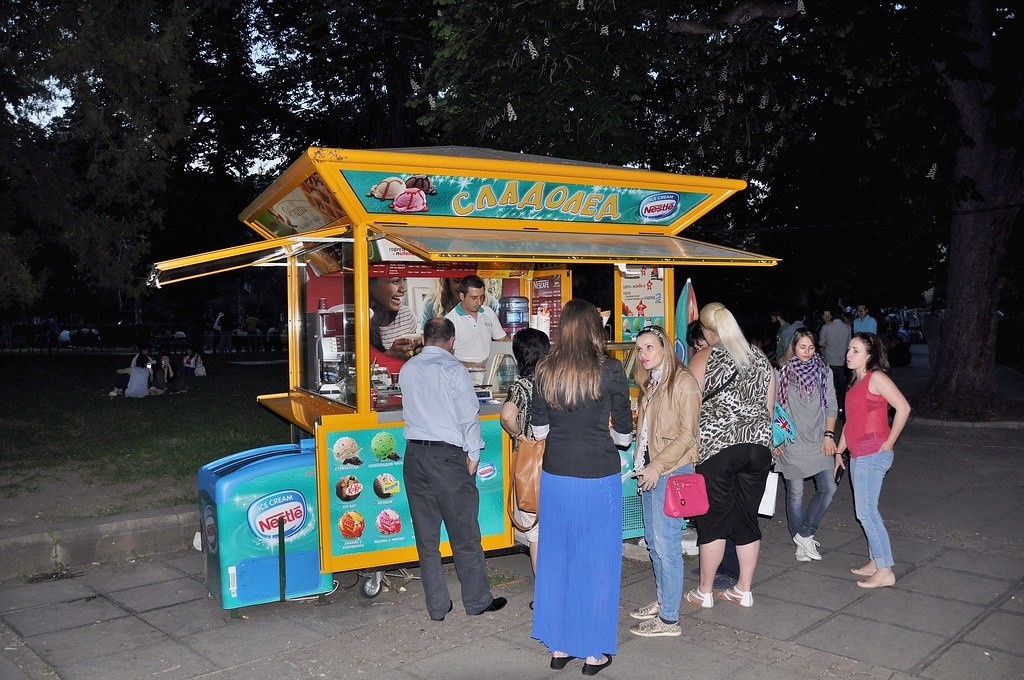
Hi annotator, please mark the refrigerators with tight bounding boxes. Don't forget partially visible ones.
[198,443,333,618]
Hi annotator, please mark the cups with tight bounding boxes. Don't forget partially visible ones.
[391,373,399,389]
[371,393,378,410]
[531,304,551,344]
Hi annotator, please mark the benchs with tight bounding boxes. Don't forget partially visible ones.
[154,333,275,356]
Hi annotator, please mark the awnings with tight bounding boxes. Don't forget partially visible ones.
[146,223,353,288]
[367,222,782,267]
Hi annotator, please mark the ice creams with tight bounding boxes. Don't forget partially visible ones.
[332,432,401,538]
[622,317,661,332]
[366,172,437,212]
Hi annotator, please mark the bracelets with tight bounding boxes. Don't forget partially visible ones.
[834,452,842,455]
[824,430,835,438]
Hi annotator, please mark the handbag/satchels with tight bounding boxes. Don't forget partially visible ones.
[505,437,548,530]
[757,470,779,517]
[663,474,715,517]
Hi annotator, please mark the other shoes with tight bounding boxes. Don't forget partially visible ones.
[474,596,510,616]
[851,567,881,577]
[549,654,579,669]
[529,601,536,612]
[581,652,613,676]
[430,599,453,621]
[857,572,896,590]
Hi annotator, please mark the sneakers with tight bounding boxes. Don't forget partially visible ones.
[629,616,681,637]
[795,545,815,562]
[628,601,663,619]
[792,533,823,561]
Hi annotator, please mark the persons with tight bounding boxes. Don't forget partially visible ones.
[529,298,633,676]
[115,343,207,398]
[499,327,554,611]
[421,278,465,346]
[819,305,852,423]
[767,305,877,371]
[173,328,185,339]
[58,326,100,351]
[211,310,261,354]
[881,305,911,369]
[398,317,508,621]
[442,274,513,386]
[368,277,425,357]
[683,302,776,610]
[773,326,839,561]
[833,331,911,589]
[629,324,704,637]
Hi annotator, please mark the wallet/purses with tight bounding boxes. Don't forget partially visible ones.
[834,458,849,485]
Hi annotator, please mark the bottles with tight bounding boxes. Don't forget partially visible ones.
[370,364,388,384]
[498,296,529,342]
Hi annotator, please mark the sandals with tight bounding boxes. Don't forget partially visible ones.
[717,586,755,607]
[684,586,716,608]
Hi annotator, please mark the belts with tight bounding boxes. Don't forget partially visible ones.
[407,439,456,449]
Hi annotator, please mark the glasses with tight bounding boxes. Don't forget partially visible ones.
[857,308,866,312]
